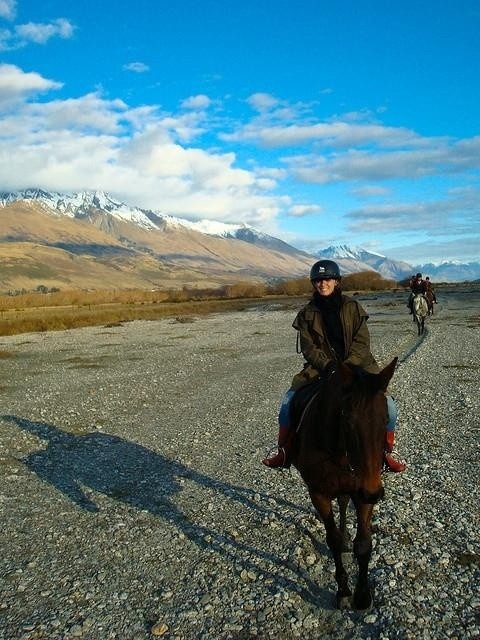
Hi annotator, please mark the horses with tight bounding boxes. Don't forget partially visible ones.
[425,286,435,316]
[289,356,398,615]
[412,295,428,337]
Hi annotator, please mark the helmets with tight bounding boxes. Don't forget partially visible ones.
[308,259,341,279]
[416,272,422,278]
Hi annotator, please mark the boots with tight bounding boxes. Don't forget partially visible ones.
[385,431,408,474]
[262,427,292,469]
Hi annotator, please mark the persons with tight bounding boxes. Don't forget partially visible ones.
[408,273,439,316]
[263,260,406,473]
